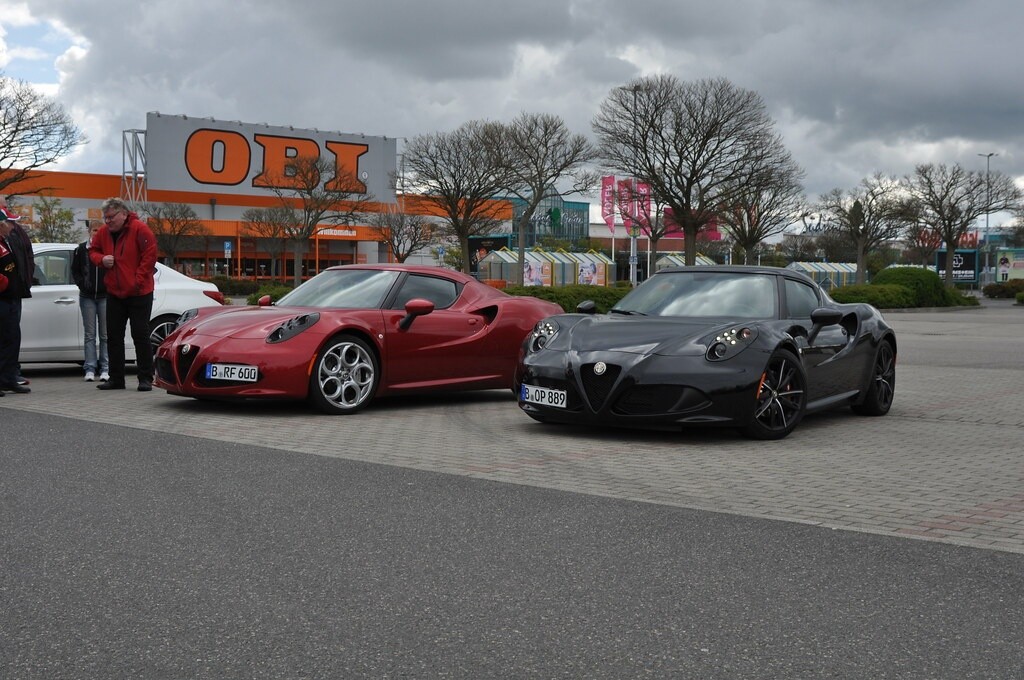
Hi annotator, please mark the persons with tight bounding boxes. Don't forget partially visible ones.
[72,220,111,382]
[88,198,156,391]
[998,253,1010,282]
[0,196,34,395]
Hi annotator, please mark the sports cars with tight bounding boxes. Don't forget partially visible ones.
[152,264,566,414]
[516,265,897,441]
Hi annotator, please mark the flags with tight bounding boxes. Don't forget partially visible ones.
[601,175,651,236]
[718,202,758,228]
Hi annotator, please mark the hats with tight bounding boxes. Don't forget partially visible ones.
[0,207,21,221]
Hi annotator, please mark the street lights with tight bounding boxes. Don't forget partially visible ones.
[619,84,650,286]
[978,152,994,287]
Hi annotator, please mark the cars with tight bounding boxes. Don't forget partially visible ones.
[20,241,225,371]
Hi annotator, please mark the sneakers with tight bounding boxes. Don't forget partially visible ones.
[16,376,29,385]
[85,372,94,381]
[99,372,110,381]
[0,382,30,394]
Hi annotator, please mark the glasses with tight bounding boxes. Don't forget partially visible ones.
[103,212,119,220]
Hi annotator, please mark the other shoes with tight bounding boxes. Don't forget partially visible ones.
[137,380,152,391]
[96,377,126,389]
